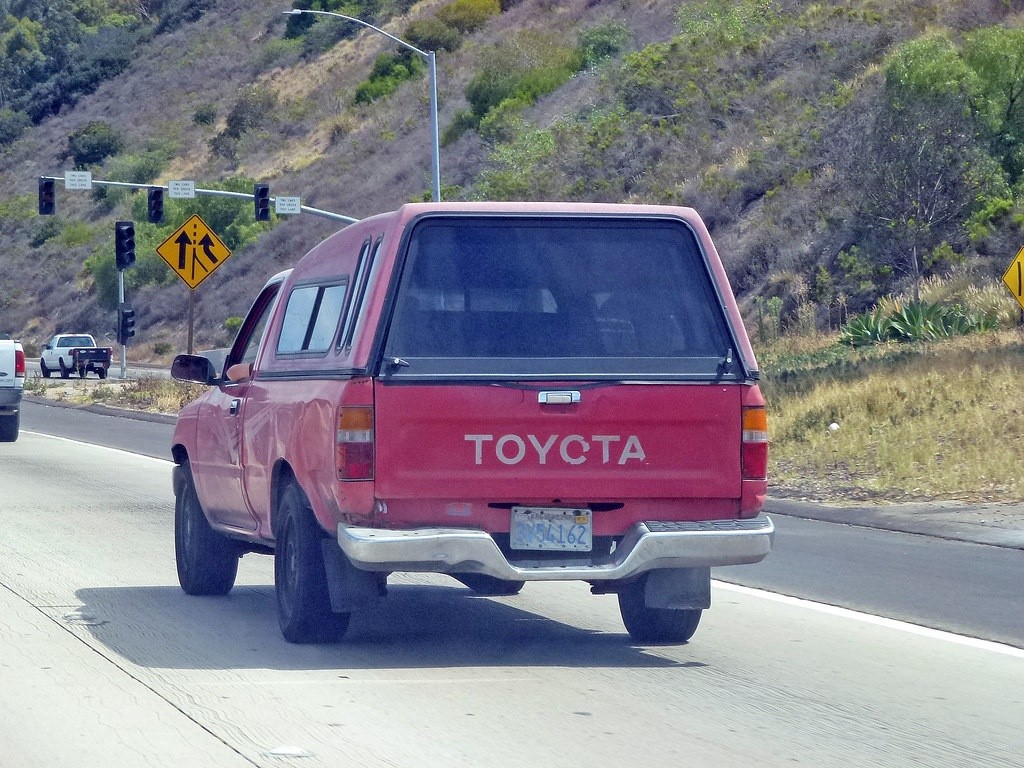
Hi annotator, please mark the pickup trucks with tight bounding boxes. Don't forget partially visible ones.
[40,333,114,379]
[0,339,27,442]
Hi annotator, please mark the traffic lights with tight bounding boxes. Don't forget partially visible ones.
[253,184,270,221]
[115,220,136,272]
[148,187,163,224]
[38,177,56,216]
[117,307,135,346]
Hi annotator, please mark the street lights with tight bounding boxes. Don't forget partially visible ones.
[280,7,441,203]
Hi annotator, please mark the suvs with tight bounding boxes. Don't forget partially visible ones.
[172,198,772,646]
